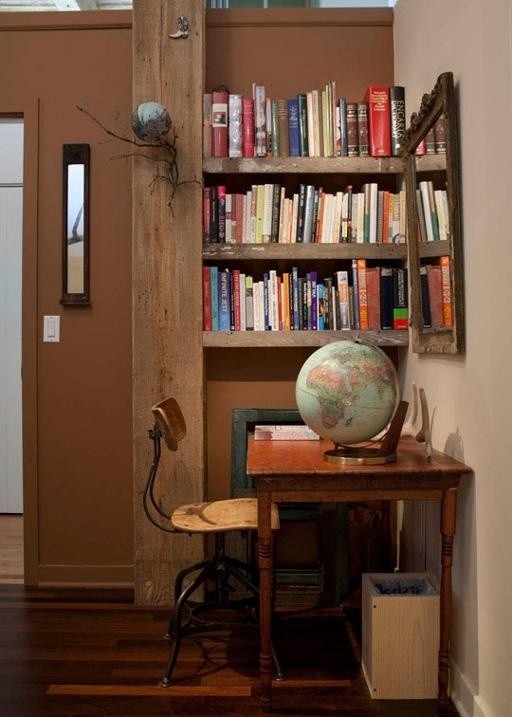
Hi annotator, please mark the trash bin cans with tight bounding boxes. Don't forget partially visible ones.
[359,571,440,700]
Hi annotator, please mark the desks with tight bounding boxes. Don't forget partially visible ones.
[243,435,474,712]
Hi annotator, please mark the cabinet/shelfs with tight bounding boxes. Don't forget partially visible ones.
[413,147,452,340]
[198,150,411,352]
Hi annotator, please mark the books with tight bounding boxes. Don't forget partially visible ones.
[203,258,408,333]
[434,113,446,153]
[204,184,407,244]
[254,424,320,441]
[415,180,452,329]
[204,82,406,158]
[424,126,436,154]
[415,138,425,155]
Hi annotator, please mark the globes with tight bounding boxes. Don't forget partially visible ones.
[295,333,409,465]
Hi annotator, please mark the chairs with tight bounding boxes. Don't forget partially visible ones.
[138,392,293,690]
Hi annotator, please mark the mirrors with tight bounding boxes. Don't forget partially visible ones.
[400,69,467,353]
